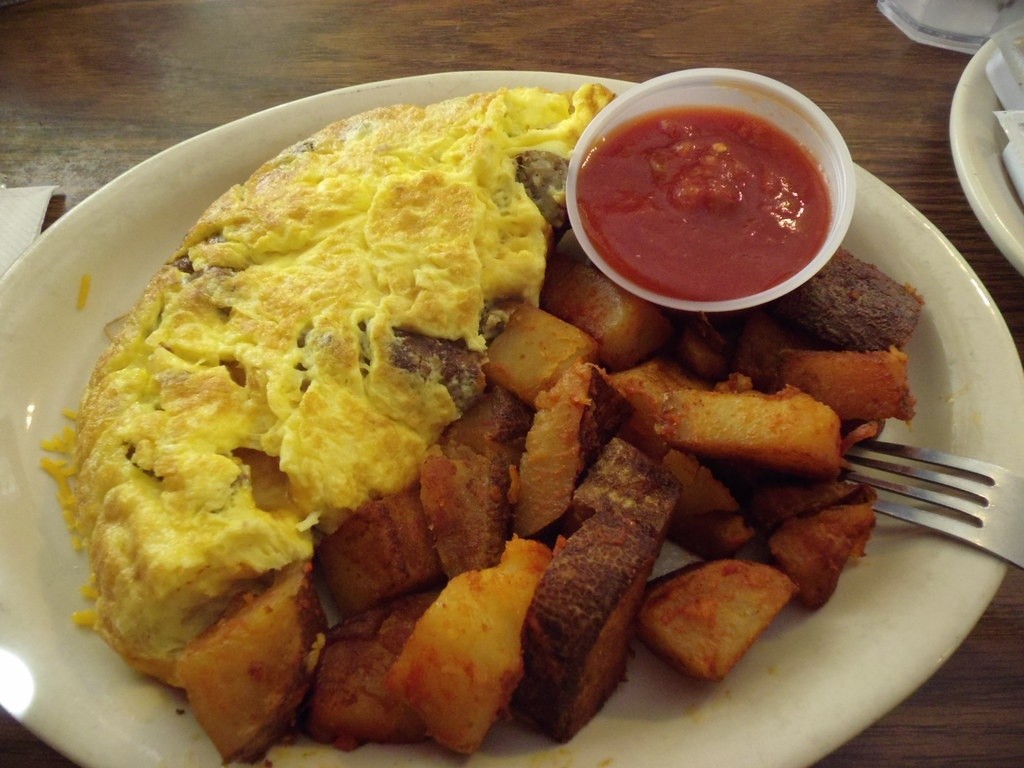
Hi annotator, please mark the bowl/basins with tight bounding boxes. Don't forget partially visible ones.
[564,67,861,314]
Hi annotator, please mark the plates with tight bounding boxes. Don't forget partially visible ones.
[0,68,1023,768]
[947,17,1023,280]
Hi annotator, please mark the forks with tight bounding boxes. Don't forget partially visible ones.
[836,438,1024,572]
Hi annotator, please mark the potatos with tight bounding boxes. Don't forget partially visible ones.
[140,246,926,768]
